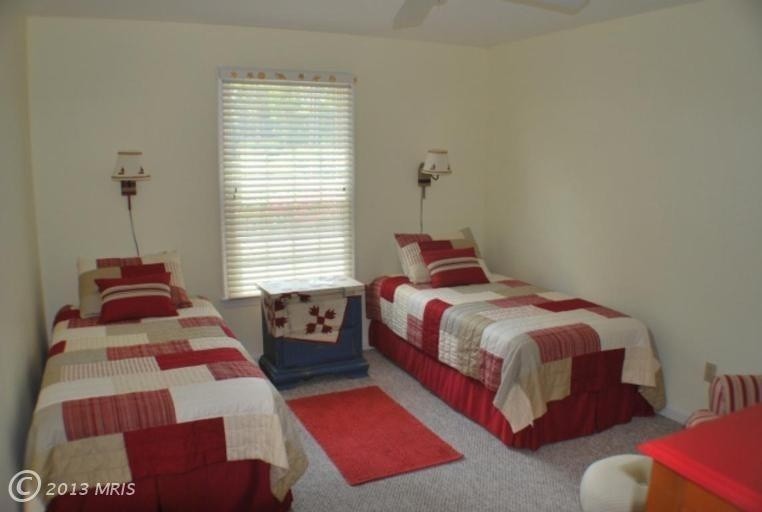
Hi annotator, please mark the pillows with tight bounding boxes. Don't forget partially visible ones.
[394,228,493,288]
[76,248,192,323]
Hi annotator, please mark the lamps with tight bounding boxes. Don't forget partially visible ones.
[416,149,454,235]
[109,151,150,257]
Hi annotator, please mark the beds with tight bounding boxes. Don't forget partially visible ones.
[363,271,668,452]
[21,296,309,512]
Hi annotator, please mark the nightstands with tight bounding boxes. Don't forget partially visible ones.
[253,273,368,392]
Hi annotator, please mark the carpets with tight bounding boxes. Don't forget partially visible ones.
[285,384,465,488]
